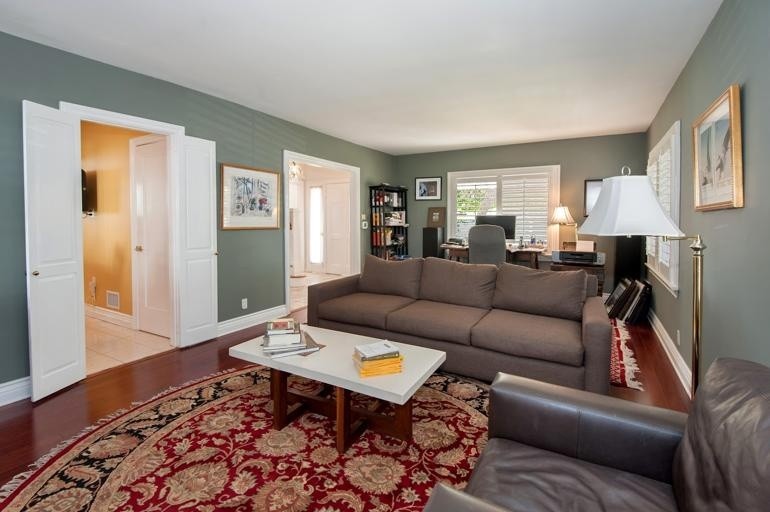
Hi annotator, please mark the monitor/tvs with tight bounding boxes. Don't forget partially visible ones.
[475,215,516,240]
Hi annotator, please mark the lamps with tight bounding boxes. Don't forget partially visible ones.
[575,165,706,399]
[549,204,575,227]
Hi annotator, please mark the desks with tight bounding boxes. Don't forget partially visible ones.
[439,241,545,269]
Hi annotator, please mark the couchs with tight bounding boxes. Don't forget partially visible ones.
[421,356,770,512]
[307,253,612,396]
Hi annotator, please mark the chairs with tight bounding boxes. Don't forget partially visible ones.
[467,224,506,264]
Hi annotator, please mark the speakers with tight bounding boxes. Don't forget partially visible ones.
[422,227,444,258]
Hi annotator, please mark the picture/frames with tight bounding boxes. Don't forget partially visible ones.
[219,162,281,230]
[690,82,745,211]
[414,176,442,201]
[582,178,604,217]
[606,277,653,324]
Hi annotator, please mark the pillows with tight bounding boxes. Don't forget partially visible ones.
[418,257,498,310]
[492,261,587,319]
[358,253,424,300]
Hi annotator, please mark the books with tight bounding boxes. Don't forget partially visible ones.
[352,340,404,378]
[259,318,328,359]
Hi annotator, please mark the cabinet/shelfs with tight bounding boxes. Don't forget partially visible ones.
[549,263,608,297]
[368,185,408,260]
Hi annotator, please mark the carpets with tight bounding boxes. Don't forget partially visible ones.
[0,359,489,512]
[609,317,644,392]
